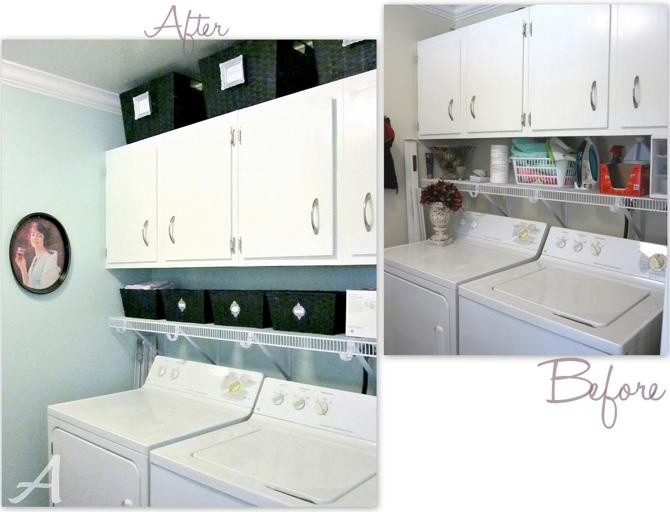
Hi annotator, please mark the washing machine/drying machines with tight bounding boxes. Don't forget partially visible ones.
[384,210,547,356]
[147,375,377,509]
[45,355,264,508]
[457,224,669,357]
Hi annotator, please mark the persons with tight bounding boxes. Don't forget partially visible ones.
[14,222,60,289]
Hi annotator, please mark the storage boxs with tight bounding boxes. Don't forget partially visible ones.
[120,288,164,319]
[160,289,213,324]
[312,39,376,84]
[207,291,271,328]
[266,291,346,335]
[198,40,316,119]
[118,72,206,144]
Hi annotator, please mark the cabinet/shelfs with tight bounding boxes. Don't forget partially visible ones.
[105,71,378,271]
[419,3,669,141]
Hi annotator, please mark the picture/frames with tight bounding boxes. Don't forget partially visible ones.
[8,212,71,295]
